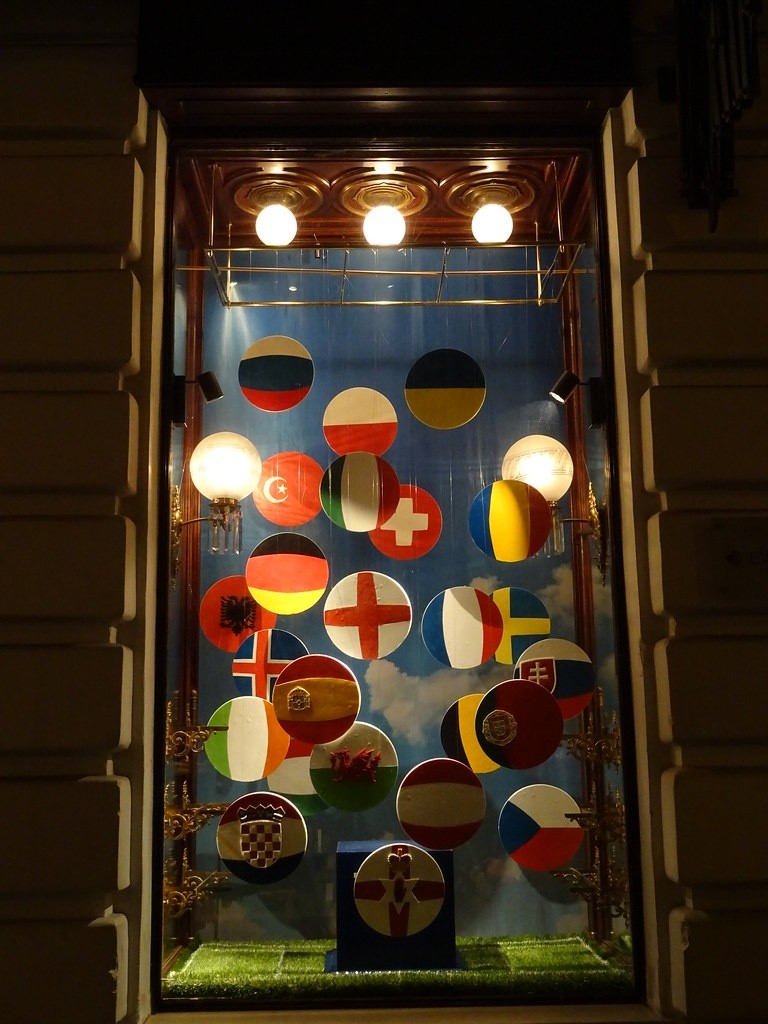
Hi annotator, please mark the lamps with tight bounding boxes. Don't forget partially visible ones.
[549,371,604,430]
[172,371,223,427]
[501,434,607,585]
[169,431,263,594]
